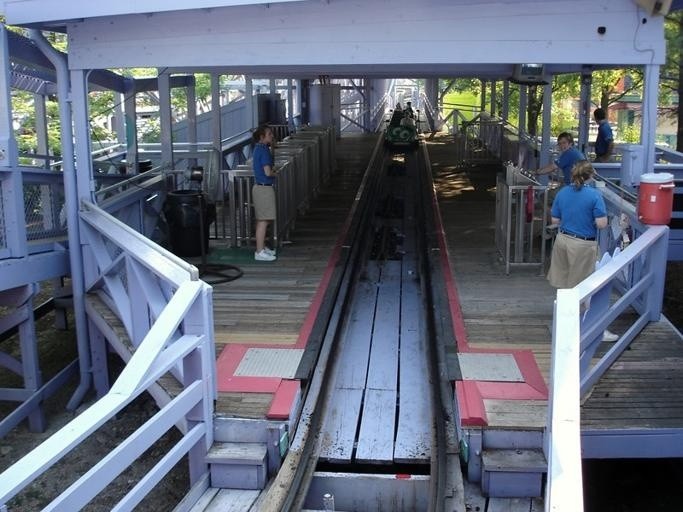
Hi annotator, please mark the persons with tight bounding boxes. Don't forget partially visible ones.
[394,102,416,127]
[546,161,620,343]
[590,108,616,162]
[529,133,589,186]
[251,126,277,263]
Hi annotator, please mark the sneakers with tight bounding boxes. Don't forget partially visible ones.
[264,246,275,255]
[601,330,618,342]
[255,249,276,261]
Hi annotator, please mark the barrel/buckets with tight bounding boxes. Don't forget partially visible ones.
[162,189,216,258]
[638,172,676,226]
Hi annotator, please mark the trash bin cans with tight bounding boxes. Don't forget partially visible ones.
[166,189,209,257]
[119,158,153,199]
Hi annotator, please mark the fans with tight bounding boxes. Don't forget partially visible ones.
[184,148,243,285]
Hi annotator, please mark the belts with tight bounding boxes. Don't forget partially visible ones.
[258,184,272,186]
[561,230,595,240]
